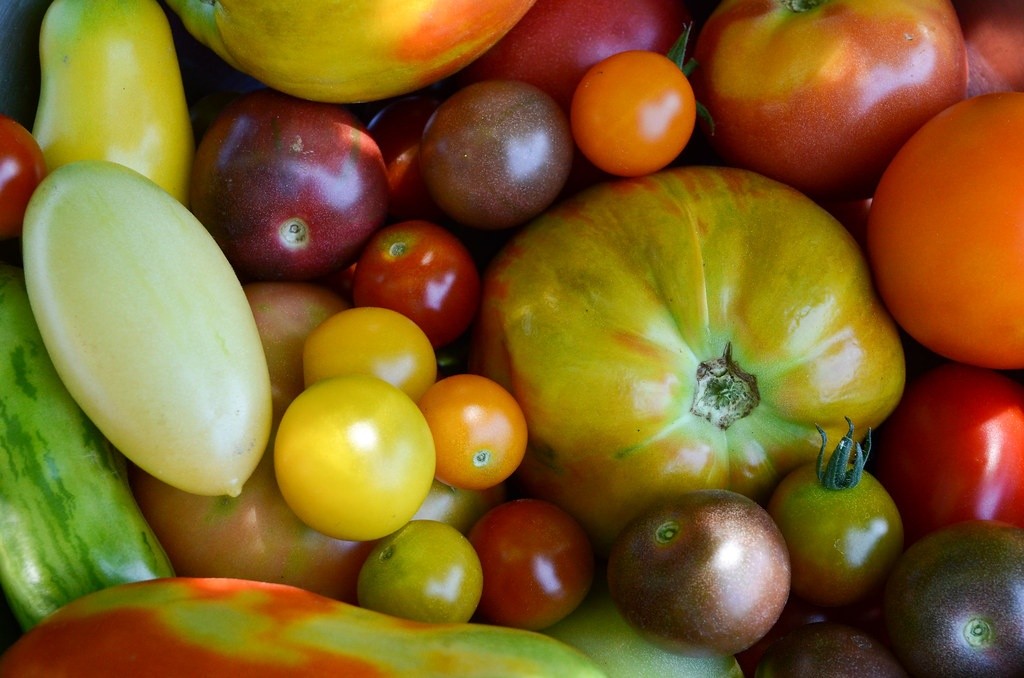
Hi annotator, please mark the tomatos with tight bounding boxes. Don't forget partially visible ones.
[0,0,1024,678]
